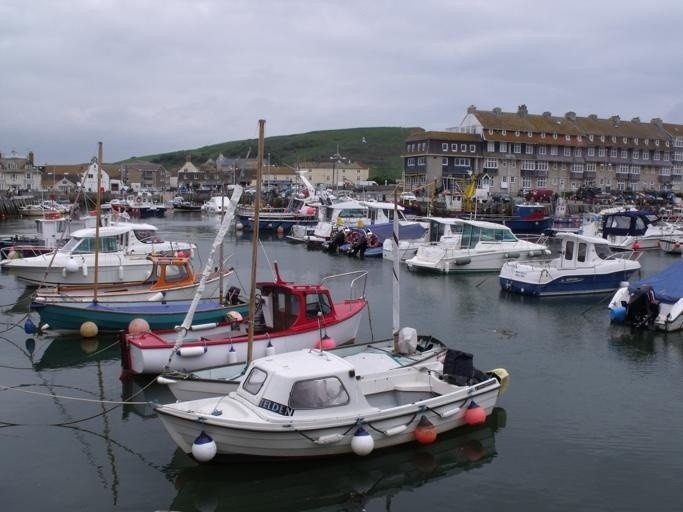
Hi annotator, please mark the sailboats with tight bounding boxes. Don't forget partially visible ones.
[153,117,512,463]
[24,141,256,338]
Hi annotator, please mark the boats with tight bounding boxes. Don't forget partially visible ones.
[0,167,200,282]
[498,202,560,238]
[116,181,372,378]
[30,240,235,305]
[497,231,641,300]
[604,251,683,335]
[581,206,682,257]
[403,216,552,275]
[199,196,232,215]
[232,178,407,260]
